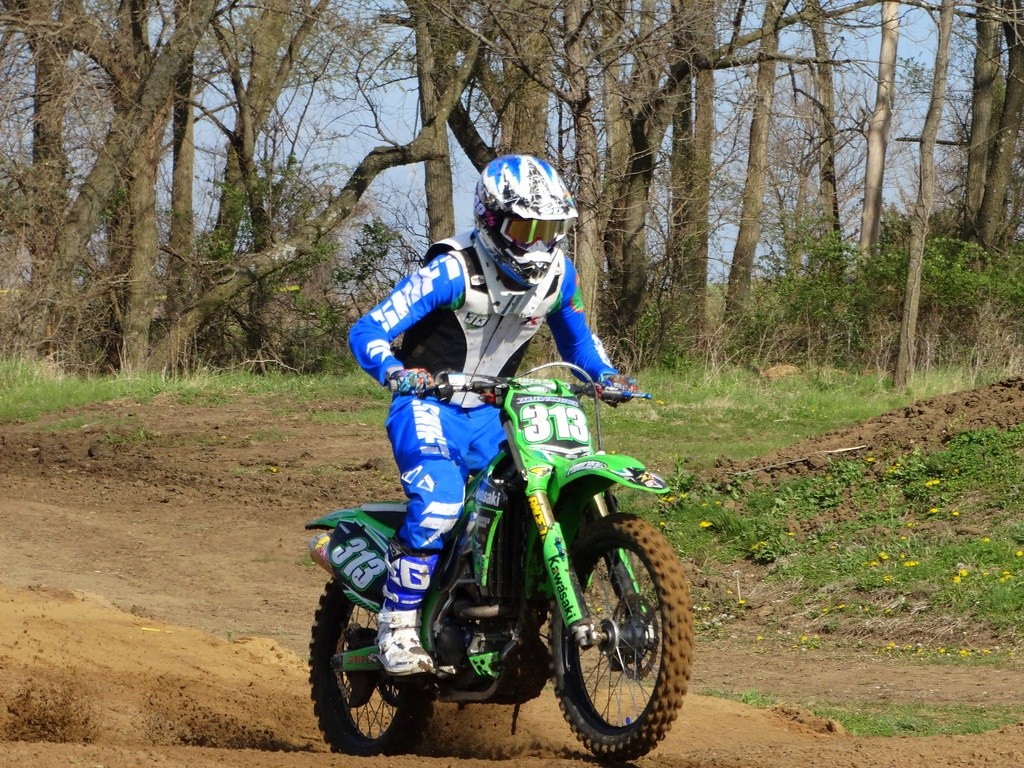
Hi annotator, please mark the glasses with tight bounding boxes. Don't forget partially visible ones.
[484,213,574,253]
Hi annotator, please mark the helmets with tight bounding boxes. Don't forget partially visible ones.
[474,154,579,288]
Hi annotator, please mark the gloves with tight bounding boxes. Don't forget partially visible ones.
[388,368,435,400]
[597,374,638,409]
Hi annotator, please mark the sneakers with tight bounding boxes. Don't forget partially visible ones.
[378,598,433,676]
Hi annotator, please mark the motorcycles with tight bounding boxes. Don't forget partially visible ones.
[307,371,696,766]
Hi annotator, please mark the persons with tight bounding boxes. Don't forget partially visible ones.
[349,154,637,677]
